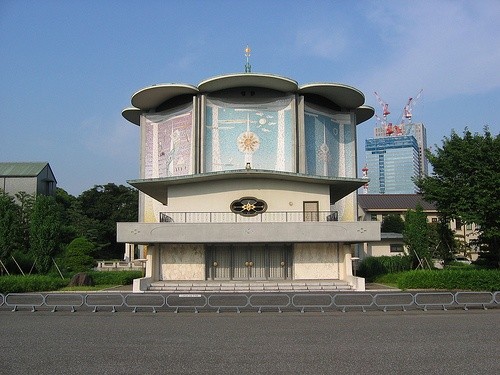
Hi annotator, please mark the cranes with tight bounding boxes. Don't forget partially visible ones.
[373,90,424,137]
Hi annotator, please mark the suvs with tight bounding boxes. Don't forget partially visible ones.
[454,254,471,264]
